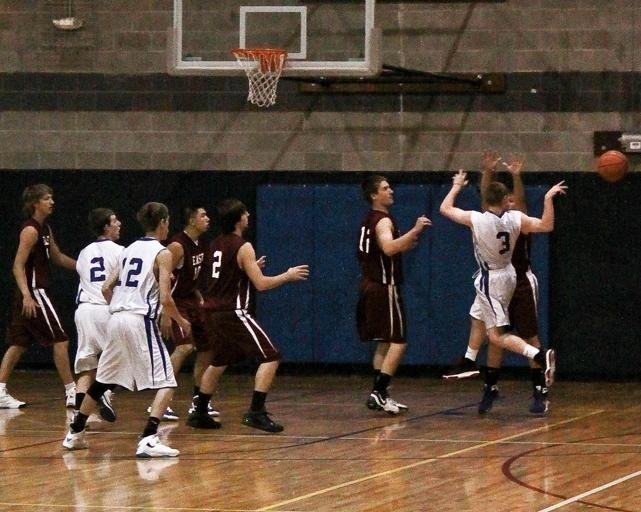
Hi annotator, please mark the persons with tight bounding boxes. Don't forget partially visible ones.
[478,148,551,417]
[185,196,309,432]
[62,201,191,457]
[356,175,432,415]
[0,184,78,410]
[439,168,569,388]
[69,208,126,426]
[146,205,220,420]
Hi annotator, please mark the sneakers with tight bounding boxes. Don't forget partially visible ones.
[368,390,408,416]
[186,397,221,416]
[0,383,27,408]
[530,393,553,414]
[99,389,117,421]
[445,363,480,381]
[239,412,285,432]
[538,349,556,385]
[134,438,182,459]
[477,385,499,415]
[64,387,79,409]
[146,401,178,420]
[63,427,91,449]
[189,412,222,429]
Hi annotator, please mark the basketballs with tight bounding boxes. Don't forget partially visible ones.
[597,150,629,183]
